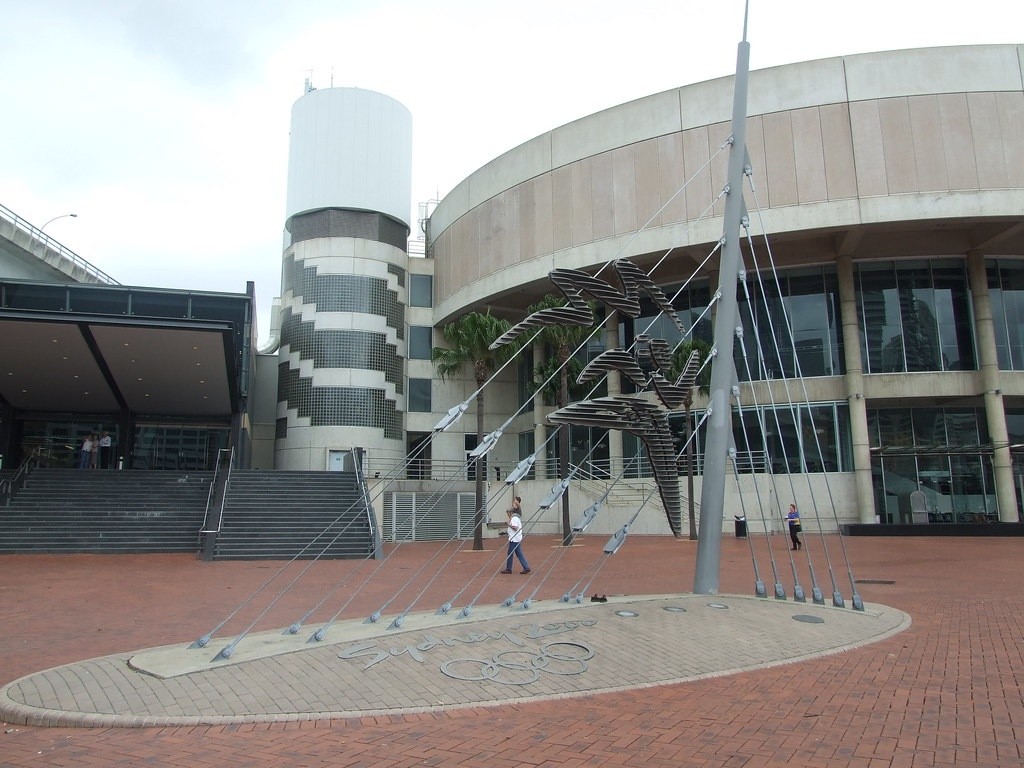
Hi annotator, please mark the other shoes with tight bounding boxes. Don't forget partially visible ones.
[790,547,797,550]
[501,569,511,574]
[520,569,530,574]
[798,543,801,550]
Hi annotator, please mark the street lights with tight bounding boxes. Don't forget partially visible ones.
[36,214,78,245]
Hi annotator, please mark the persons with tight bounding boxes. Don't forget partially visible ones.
[500,497,530,574]
[80,432,112,469]
[785,504,801,550]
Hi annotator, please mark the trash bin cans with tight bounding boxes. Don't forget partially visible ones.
[735,515,747,539]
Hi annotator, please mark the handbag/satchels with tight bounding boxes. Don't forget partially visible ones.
[794,524,802,532]
[79,440,86,450]
